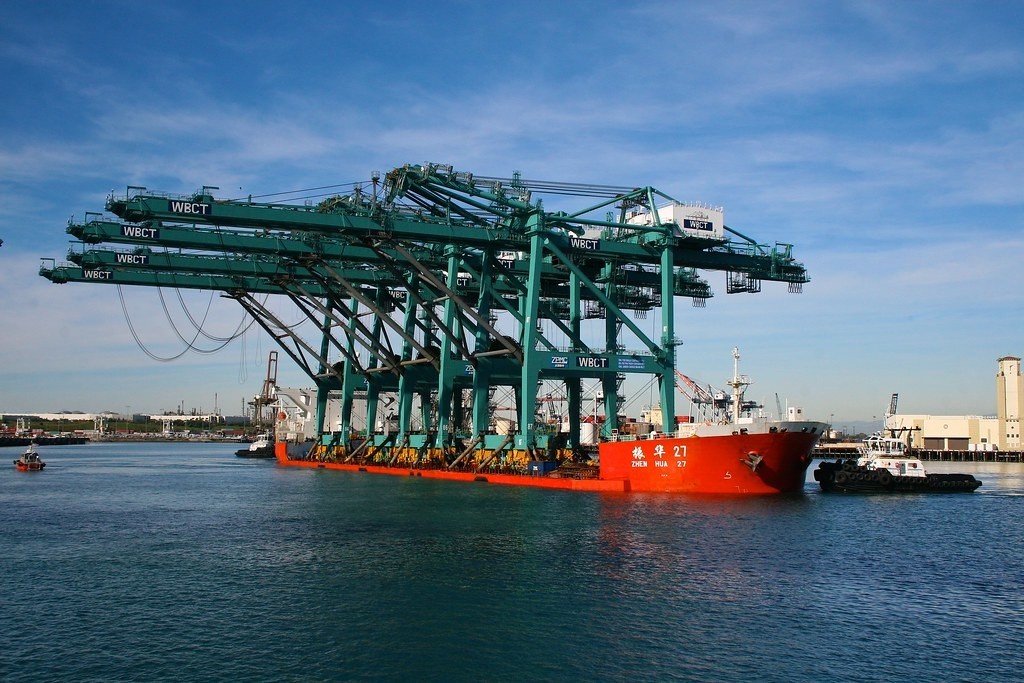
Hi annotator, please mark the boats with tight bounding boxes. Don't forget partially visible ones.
[236,433,276,457]
[14,442,45,472]
[814,427,982,494]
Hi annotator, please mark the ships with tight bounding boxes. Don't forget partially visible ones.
[38,163,830,498]
